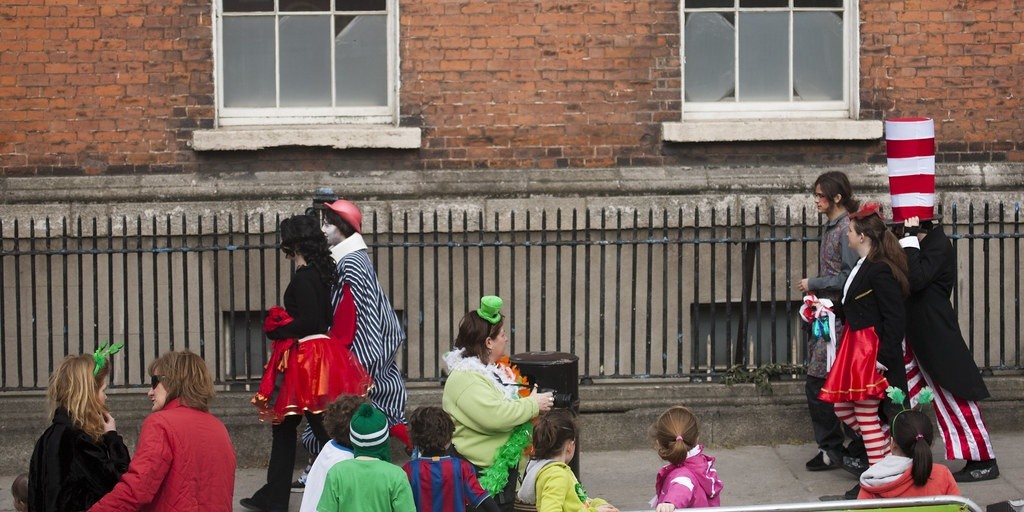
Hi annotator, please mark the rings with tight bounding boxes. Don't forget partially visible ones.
[549,398,553,403]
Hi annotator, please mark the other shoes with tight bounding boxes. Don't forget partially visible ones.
[951,462,1000,482]
[240,498,260,510]
[846,483,861,500]
[806,451,839,470]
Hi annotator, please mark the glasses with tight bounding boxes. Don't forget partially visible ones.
[152,375,166,389]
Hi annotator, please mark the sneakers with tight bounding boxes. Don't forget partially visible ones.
[292,473,307,492]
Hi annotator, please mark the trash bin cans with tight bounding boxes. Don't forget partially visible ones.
[510,352,580,483]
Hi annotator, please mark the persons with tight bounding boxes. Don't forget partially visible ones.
[798,170,861,471]
[402,406,499,512]
[441,295,554,512]
[299,394,369,512]
[517,411,621,512]
[817,202,912,500]
[316,402,416,512]
[238,207,376,512]
[26,340,131,512]
[855,410,962,499]
[885,198,1000,483]
[12,473,30,512]
[86,350,236,511]
[647,406,724,512]
[290,199,412,493]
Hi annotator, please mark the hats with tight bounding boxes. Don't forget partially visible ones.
[350,403,391,461]
[324,200,361,233]
[885,117,943,225]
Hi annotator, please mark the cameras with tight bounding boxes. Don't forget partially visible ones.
[530,385,573,409]
